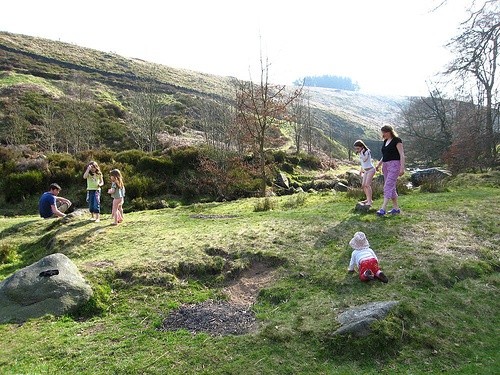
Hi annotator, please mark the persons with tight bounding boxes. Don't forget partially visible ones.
[353,140,376,206]
[83,161,104,223]
[375,125,405,216]
[107,169,125,225]
[348,232,388,283]
[38,183,72,219]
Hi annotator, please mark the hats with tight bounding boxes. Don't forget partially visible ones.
[349,232,369,249]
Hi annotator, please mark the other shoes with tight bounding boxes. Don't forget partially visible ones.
[364,269,374,281]
[90,218,96,220]
[95,219,100,222]
[377,270,388,283]
[361,200,368,203]
[111,222,117,224]
[118,219,123,223]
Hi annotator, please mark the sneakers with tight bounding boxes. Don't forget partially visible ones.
[376,209,386,215]
[387,208,400,214]
[366,200,373,205]
